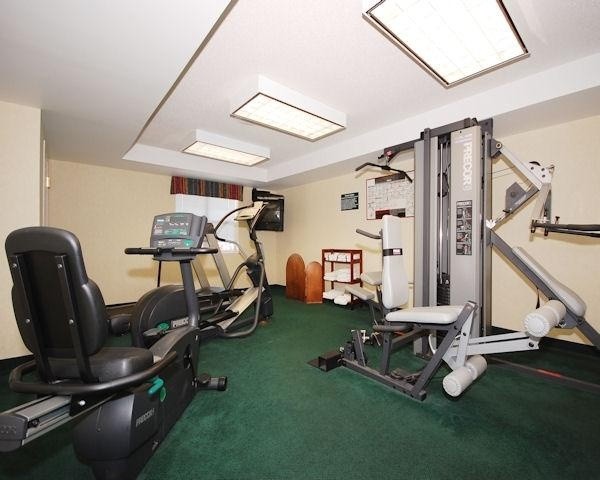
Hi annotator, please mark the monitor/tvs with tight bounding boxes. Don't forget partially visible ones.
[254,198,284,232]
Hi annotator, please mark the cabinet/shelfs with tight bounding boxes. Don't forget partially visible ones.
[322,248,363,309]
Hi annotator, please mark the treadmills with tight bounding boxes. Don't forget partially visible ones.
[105,224,237,339]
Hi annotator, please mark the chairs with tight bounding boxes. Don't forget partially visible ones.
[4,227,152,382]
[382,213,466,325]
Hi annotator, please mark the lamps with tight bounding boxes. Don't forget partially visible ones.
[361,0,530,89]
[228,74,347,144]
[181,130,272,168]
